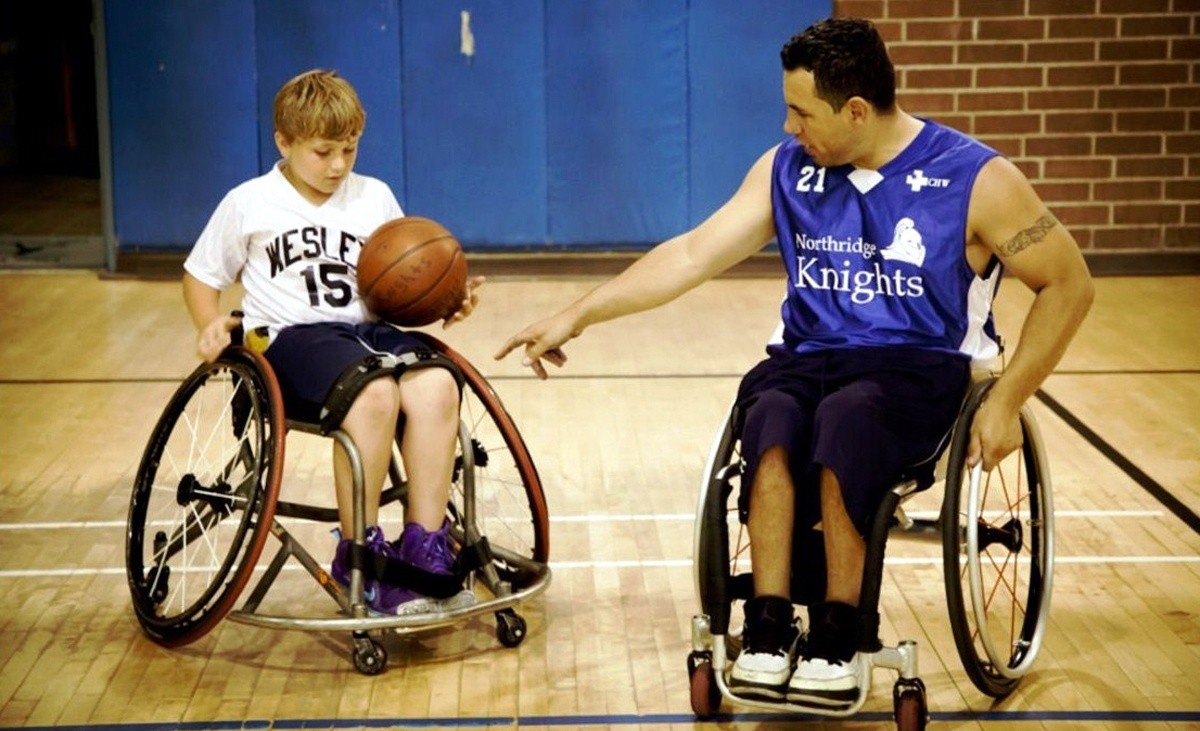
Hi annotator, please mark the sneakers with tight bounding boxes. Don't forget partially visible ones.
[332,525,426,615]
[787,602,863,704]
[732,595,801,686]
[388,518,473,610]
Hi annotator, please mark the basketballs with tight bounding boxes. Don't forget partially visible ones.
[357,216,466,330]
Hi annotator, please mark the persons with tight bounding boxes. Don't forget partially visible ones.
[183,68,488,617]
[493,15,1098,718]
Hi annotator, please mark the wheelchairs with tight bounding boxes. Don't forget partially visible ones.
[686,332,1056,731]
[124,310,552,675]
